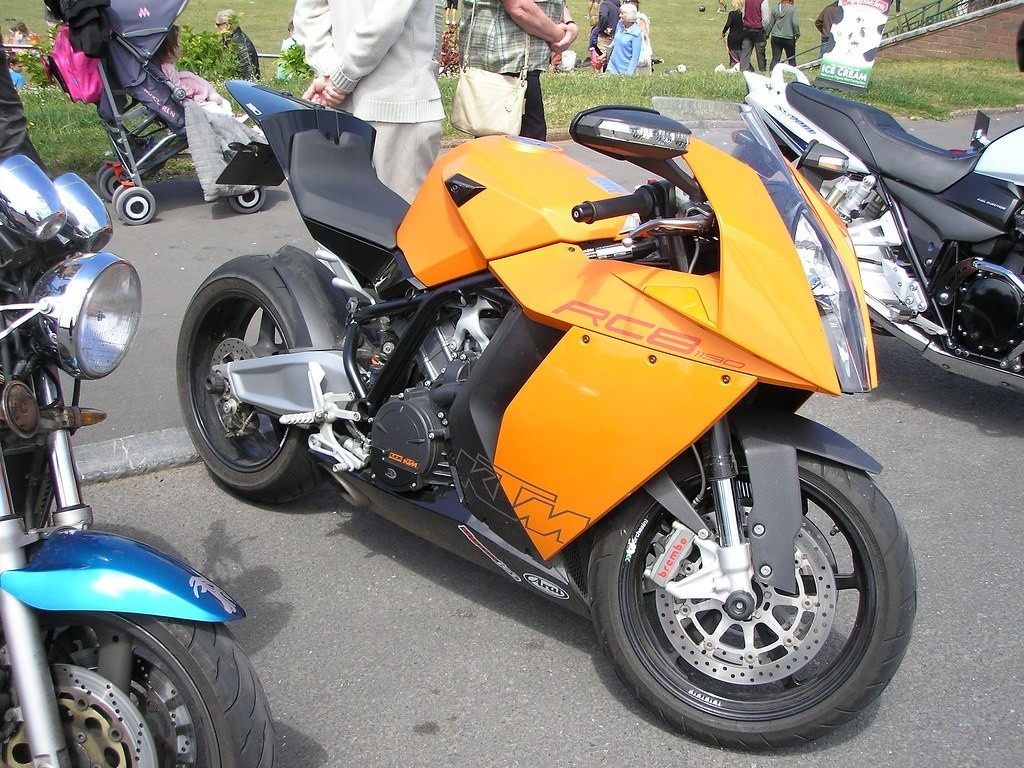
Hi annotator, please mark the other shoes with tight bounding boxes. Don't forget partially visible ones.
[446,20,450,24]
[452,21,456,25]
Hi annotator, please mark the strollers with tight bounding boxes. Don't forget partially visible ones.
[44,1,286,226]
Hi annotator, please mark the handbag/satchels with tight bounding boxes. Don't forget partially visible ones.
[451,66,528,136]
[43,26,103,106]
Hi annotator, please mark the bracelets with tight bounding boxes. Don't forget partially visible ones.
[566,21,575,25]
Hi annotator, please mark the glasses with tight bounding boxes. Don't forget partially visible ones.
[216,22,227,27]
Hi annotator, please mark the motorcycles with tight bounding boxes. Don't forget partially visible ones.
[175,79,920,753]
[731,62,1024,395]
[0,154,280,768]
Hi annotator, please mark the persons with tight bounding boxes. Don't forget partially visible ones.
[293,0,446,208]
[457,0,579,141]
[6,21,40,89]
[587,0,653,75]
[153,25,268,146]
[721,0,801,71]
[215,9,260,82]
[890,0,901,16]
[276,17,295,79]
[815,0,839,59]
